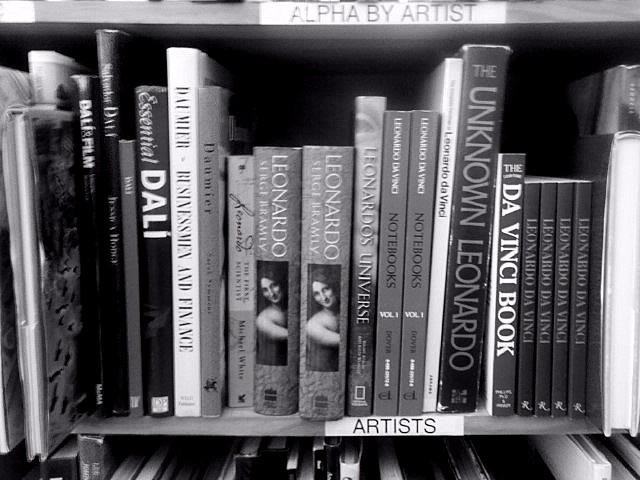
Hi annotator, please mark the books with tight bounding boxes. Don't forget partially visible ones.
[0,23,640,480]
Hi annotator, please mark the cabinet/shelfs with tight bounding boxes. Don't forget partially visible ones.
[1,0,639,479]
